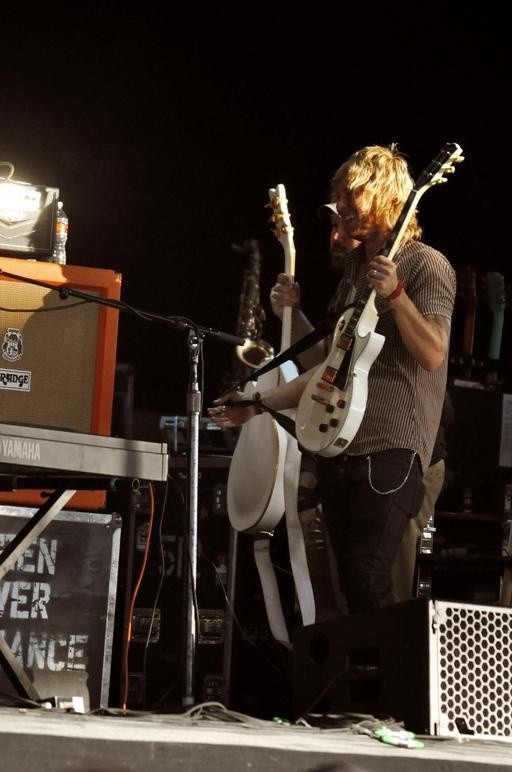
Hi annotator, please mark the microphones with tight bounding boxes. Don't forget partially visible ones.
[217,331,245,346]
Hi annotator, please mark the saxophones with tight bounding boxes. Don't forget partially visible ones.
[231,239,274,390]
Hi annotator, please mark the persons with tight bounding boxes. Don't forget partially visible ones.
[206,143,460,612]
[270,197,446,601]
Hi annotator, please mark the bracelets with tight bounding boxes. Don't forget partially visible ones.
[385,282,407,303]
[253,392,262,414]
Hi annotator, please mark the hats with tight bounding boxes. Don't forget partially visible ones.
[321,201,342,219]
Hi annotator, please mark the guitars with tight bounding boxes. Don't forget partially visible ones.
[296,140,464,457]
[226,182,300,533]
